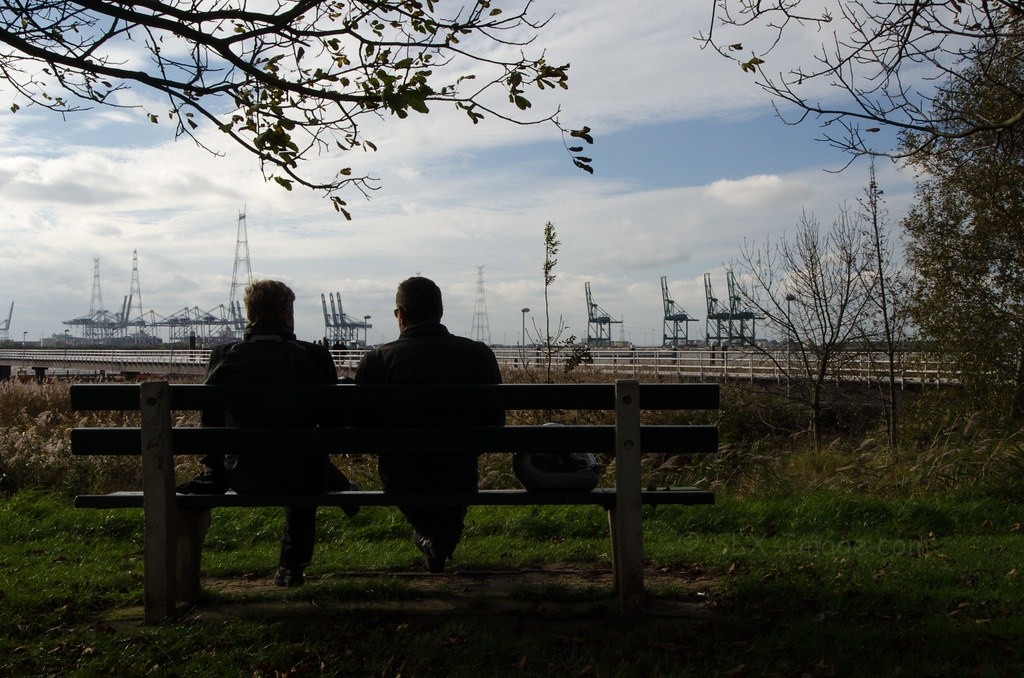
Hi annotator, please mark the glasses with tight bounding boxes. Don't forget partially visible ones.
[394,308,399,316]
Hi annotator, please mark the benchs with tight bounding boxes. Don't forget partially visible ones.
[67,378,721,628]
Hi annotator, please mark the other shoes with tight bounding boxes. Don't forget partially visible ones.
[413,532,449,571]
[273,566,305,588]
[341,479,359,517]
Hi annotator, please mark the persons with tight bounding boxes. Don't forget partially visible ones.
[200,282,359,591]
[361,278,505,577]
